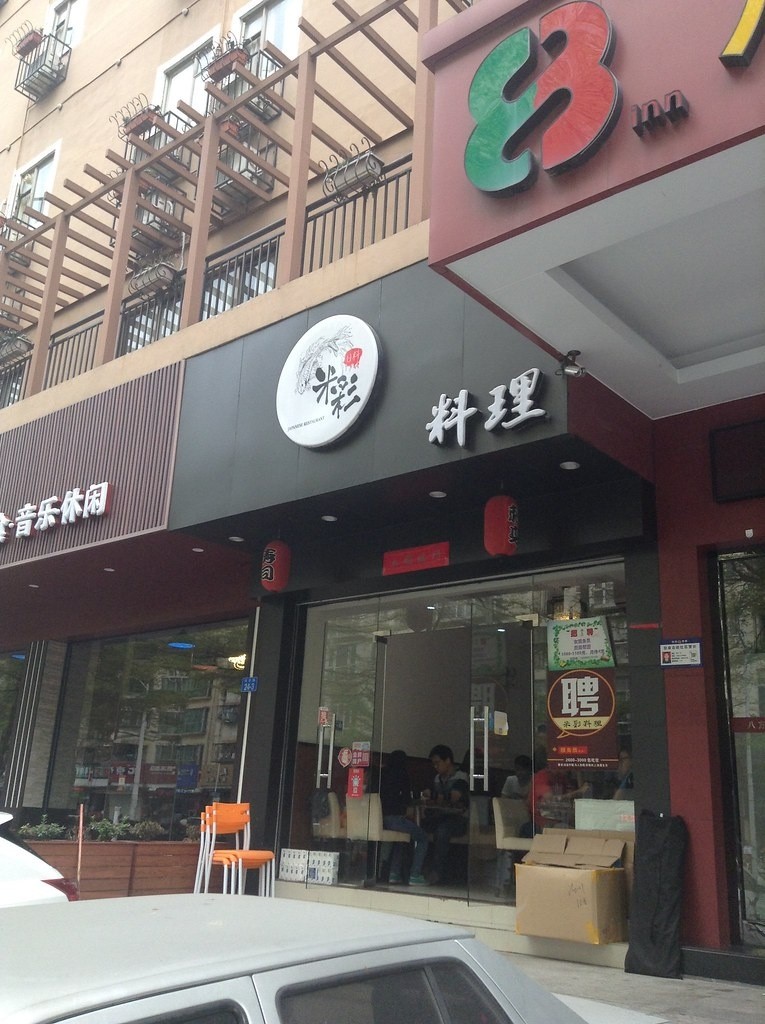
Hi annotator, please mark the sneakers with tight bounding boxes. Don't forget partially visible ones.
[389,874,406,883]
[409,875,430,886]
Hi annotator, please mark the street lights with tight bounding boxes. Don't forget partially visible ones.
[116,673,150,827]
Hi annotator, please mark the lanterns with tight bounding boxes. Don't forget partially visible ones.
[261,539,291,592]
[484,495,520,558]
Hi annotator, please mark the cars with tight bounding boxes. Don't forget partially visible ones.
[0,812,81,907]
[0,893,590,1023]
[180,817,229,842]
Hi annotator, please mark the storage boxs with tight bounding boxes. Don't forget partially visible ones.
[514,862,629,945]
[279,848,340,886]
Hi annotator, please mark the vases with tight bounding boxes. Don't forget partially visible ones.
[15,30,44,58]
[133,262,177,295]
[205,46,250,83]
[124,107,160,138]
[197,118,240,147]
[113,170,157,202]
[328,152,385,197]
[0,336,34,364]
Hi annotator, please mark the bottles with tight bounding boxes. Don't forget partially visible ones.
[406,790,426,820]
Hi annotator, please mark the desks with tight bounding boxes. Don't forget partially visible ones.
[408,799,465,887]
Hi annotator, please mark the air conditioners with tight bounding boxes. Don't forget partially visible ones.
[0,228,34,275]
[131,190,174,258]
[213,141,261,211]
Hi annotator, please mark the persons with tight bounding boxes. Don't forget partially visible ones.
[365,725,633,886]
[663,652,671,663]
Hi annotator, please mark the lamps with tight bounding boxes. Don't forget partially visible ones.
[547,586,588,620]
[228,653,246,670]
[167,627,196,649]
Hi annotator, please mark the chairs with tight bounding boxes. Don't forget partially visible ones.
[195,801,275,898]
[311,791,411,888]
[426,797,534,898]
